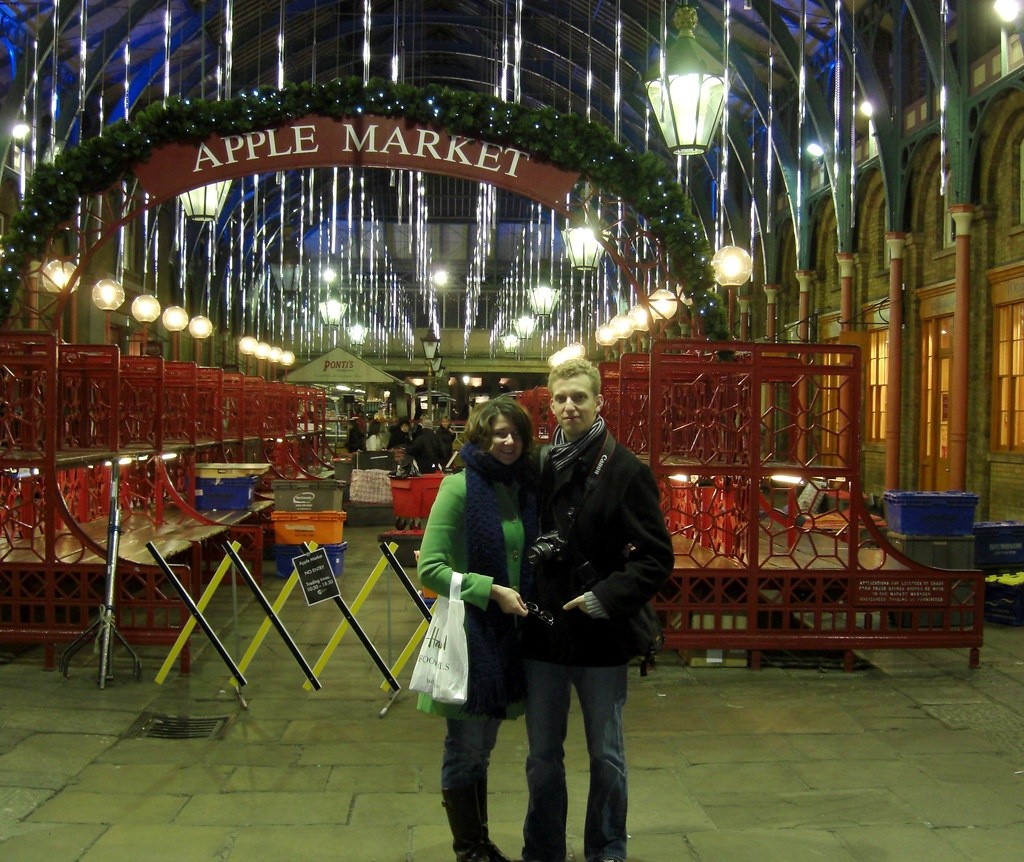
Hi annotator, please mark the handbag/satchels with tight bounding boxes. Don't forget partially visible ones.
[409,571,469,705]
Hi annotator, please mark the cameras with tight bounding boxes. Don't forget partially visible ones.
[526,529,569,566]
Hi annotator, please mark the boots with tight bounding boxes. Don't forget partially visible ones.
[439,786,520,862]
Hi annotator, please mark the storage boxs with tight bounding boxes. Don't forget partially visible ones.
[882,489,1024,629]
[269,480,349,579]
[184,476,259,511]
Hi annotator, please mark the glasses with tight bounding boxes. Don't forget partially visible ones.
[523,599,556,628]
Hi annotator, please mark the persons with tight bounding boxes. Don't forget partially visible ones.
[418,396,534,862]
[518,357,674,862]
[347,414,457,474]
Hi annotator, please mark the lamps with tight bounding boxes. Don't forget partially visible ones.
[189,315,213,366]
[608,312,633,356]
[280,350,295,383]
[345,311,369,347]
[595,323,616,362]
[269,172,309,297]
[420,284,446,380]
[93,279,124,344]
[170,1,234,223]
[643,0,737,157]
[132,294,160,356]
[626,304,651,353]
[499,324,521,355]
[43,259,80,343]
[240,336,258,377]
[648,289,678,338]
[254,342,271,376]
[675,275,717,337]
[713,244,752,337]
[560,201,612,272]
[318,287,347,327]
[267,345,283,381]
[527,212,561,315]
[163,306,189,361]
[512,306,539,340]
